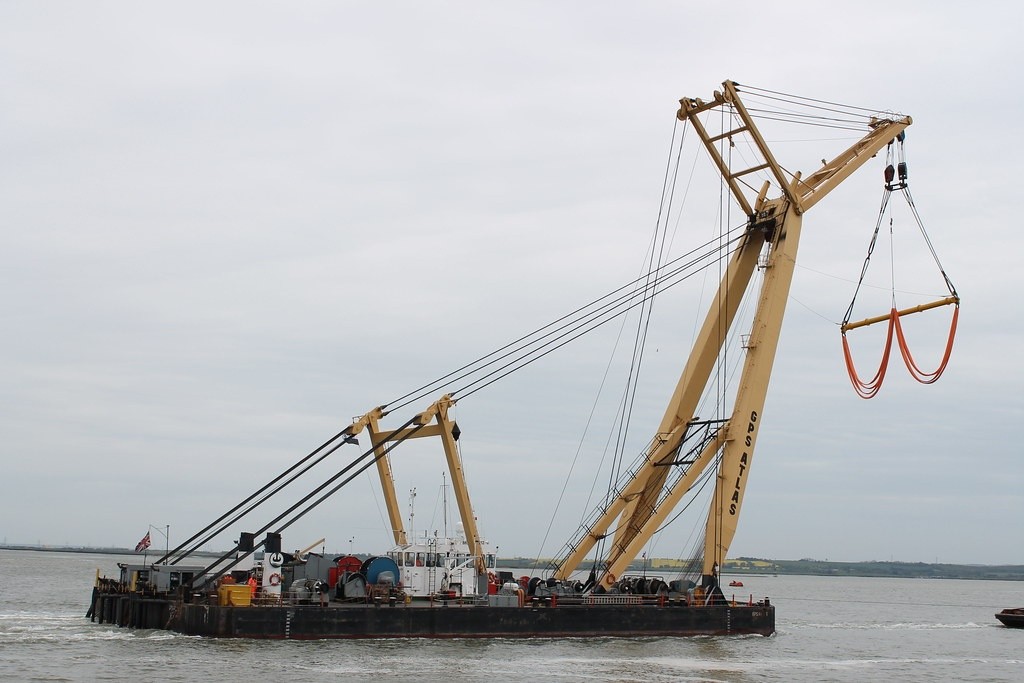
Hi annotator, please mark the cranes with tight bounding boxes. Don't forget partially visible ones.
[151,77,963,597]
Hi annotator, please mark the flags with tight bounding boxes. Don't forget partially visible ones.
[135,532,151,552]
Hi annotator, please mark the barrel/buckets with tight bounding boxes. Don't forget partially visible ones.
[488,583,496,595]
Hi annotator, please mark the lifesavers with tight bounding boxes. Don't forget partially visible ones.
[607,574,615,585]
[269,573,281,586]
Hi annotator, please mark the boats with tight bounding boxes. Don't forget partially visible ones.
[82,531,777,643]
[994,607,1024,629]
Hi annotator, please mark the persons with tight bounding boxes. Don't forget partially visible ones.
[227,574,232,579]
[248,574,258,599]
[416,558,421,566]
